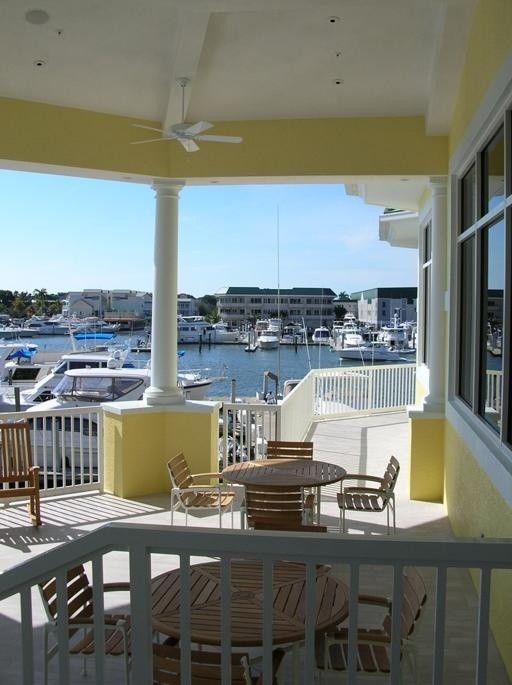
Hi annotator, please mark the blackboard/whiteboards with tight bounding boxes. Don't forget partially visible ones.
[12,368,41,380]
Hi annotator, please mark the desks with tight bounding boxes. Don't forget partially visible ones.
[148,560,352,685]
[222,459,346,526]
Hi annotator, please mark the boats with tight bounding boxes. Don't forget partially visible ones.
[486,322,503,357]
[0,351,215,411]
[1,368,358,470]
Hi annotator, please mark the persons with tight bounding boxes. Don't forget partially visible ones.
[266,390,276,404]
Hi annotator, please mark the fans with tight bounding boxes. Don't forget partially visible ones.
[131,78,243,152]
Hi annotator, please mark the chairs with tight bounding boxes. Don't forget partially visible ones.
[316,564,427,683]
[143,638,252,684]
[39,563,130,683]
[241,484,315,529]
[244,522,332,559]
[267,441,329,505]
[167,452,235,529]
[0,423,41,526]
[337,456,399,535]
